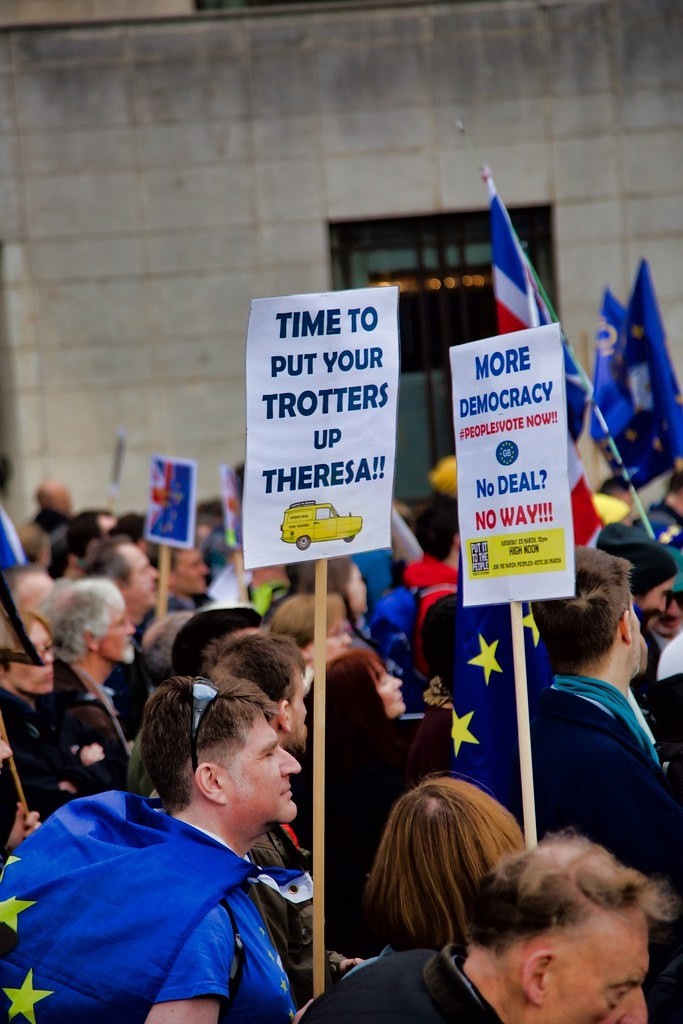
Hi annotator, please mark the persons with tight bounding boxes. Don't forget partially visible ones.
[0,458,683,1024]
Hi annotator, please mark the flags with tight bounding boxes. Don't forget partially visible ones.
[450,178,683,806]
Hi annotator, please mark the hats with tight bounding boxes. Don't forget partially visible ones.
[597,521,677,596]
[426,455,458,500]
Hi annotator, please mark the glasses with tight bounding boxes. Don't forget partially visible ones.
[189,676,220,772]
